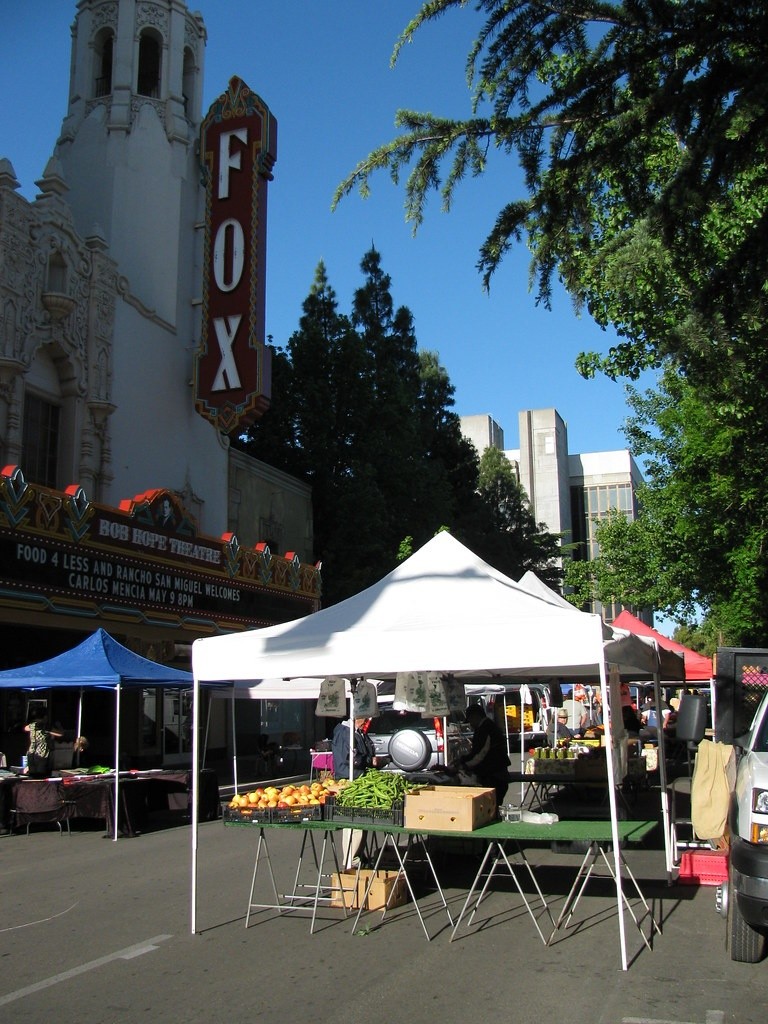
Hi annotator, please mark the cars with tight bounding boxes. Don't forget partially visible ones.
[677,674,767,964]
[625,679,712,719]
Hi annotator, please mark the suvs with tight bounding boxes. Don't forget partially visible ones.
[361,703,473,782]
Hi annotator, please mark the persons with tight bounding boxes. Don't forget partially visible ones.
[156,497,176,529]
[332,718,389,869]
[546,709,570,746]
[455,704,511,813]
[563,685,603,735]
[639,687,672,728]
[25,707,54,772]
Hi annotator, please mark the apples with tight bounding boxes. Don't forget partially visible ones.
[227,778,347,817]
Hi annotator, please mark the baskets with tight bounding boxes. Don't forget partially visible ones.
[324,796,404,826]
[221,785,324,824]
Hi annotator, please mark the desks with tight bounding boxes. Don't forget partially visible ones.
[519,756,647,816]
[9,766,218,837]
[225,817,660,972]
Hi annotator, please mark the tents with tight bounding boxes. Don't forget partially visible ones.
[192,532,716,971]
[0,626,235,839]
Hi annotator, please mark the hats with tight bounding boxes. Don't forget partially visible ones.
[558,709,571,718]
[463,704,481,723]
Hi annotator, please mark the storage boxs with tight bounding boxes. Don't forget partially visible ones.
[220,802,270,823]
[496,703,534,733]
[679,850,728,885]
[325,796,404,826]
[404,784,495,832]
[270,804,323,824]
[331,869,406,911]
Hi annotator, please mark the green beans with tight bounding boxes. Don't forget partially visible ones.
[337,767,423,808]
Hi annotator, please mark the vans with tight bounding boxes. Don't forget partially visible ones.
[496,684,555,754]
